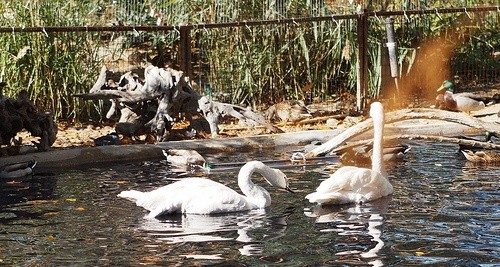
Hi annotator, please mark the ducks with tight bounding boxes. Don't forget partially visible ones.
[456,131,496,154]
[437,79,498,118]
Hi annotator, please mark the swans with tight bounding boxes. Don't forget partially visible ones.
[304,101,395,205]
[115,160,296,221]
